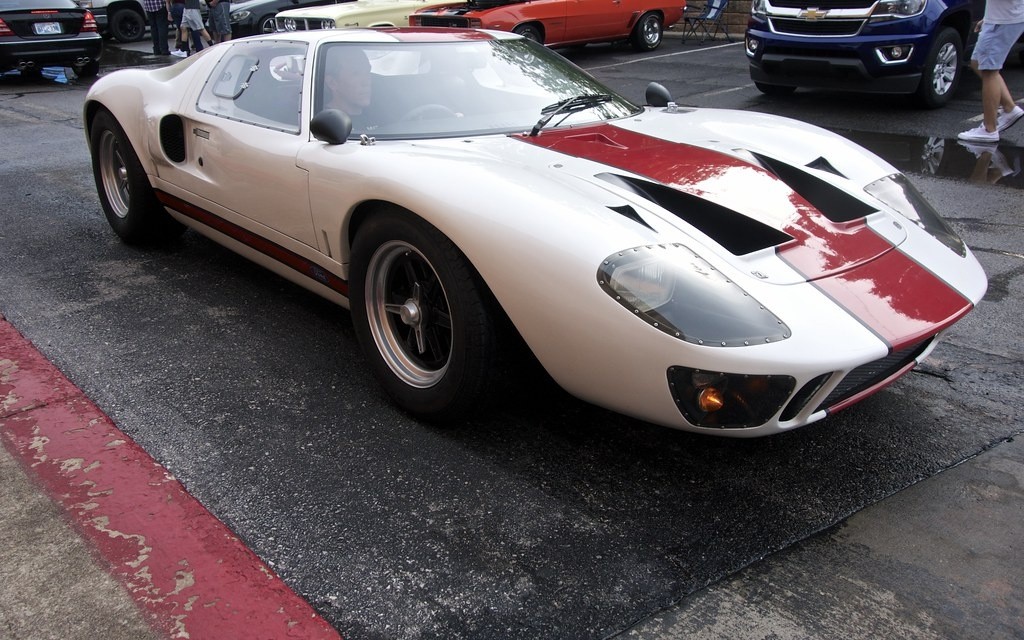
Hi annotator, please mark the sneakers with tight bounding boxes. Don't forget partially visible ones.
[958,122,999,143]
[996,105,1024,132]
[170,49,187,57]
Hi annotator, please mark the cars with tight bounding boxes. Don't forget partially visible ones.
[204,0,358,47]
[409,0,687,52]
[0,0,103,78]
[80,0,211,42]
[274,0,472,36]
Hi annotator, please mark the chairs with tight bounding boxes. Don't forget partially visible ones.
[416,49,507,114]
[681,0,735,47]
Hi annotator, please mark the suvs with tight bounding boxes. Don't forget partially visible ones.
[743,0,1024,108]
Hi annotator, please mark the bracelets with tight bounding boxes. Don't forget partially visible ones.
[167,11,171,12]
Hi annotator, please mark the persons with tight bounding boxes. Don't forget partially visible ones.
[165,0,203,56]
[204,0,232,44]
[143,0,171,55]
[957,140,1014,185]
[958,0,1024,141]
[328,48,464,130]
[174,28,196,55]
[170,0,214,58]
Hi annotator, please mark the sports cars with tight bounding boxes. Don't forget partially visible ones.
[82,22,991,439]
